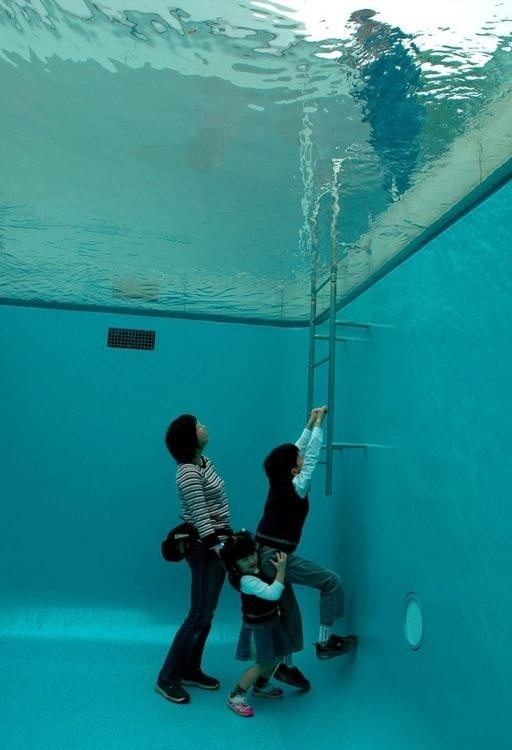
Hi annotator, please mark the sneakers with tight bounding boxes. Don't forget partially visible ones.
[274,663,310,689]
[251,683,283,698]
[227,693,253,717]
[316,635,357,659]
[155,680,190,703]
[180,669,219,690]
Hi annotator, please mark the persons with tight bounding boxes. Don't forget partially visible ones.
[221,530,288,716]
[153,415,231,703]
[253,405,357,690]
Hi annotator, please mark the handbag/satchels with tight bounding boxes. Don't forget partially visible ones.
[162,523,199,562]
